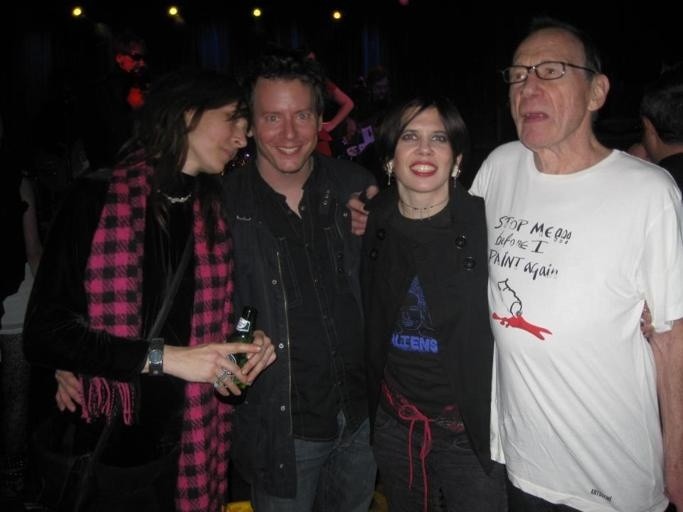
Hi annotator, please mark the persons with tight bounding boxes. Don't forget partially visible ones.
[2,17,683,512]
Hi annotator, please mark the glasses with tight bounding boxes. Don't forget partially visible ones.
[502,61,595,84]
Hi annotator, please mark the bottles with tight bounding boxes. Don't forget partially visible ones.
[213,305,258,405]
